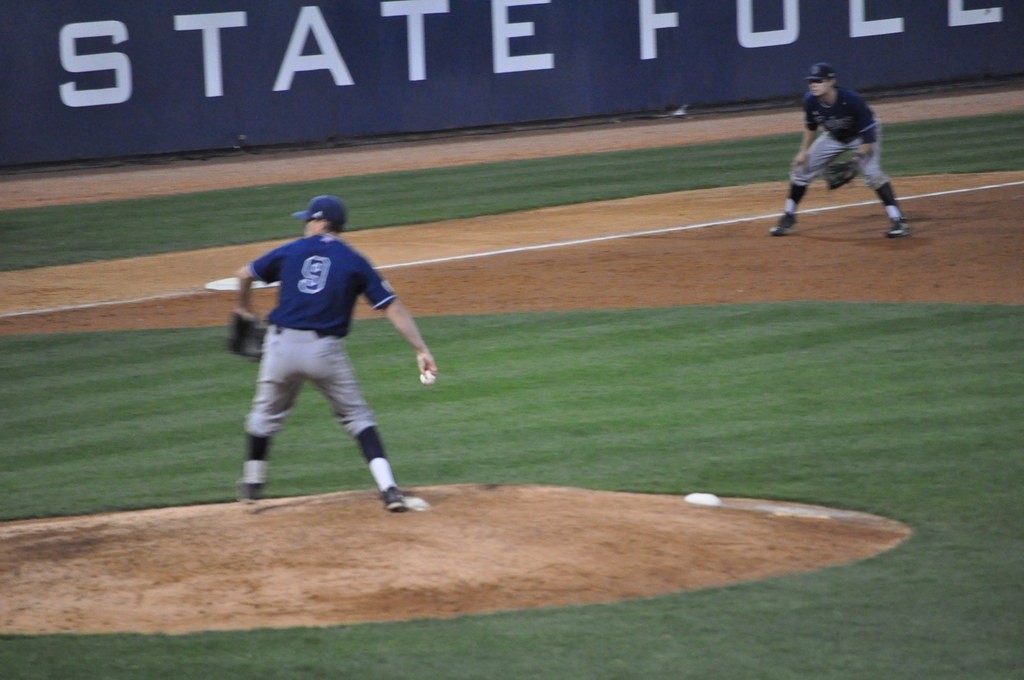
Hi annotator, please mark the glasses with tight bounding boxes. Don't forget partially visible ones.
[808,79,826,84]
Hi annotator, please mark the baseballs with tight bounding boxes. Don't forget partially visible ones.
[420,369,436,385]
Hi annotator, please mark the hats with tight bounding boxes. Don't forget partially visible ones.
[291,196,346,224]
[803,63,836,80]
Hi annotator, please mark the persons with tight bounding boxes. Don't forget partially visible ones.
[768,63,912,238]
[234,196,437,514]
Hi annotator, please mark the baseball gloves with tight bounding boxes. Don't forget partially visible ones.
[822,160,859,190]
[228,307,267,363]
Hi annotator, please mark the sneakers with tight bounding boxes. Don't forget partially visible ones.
[769,214,796,235]
[378,487,407,510]
[886,217,910,237]
[233,482,257,505]
